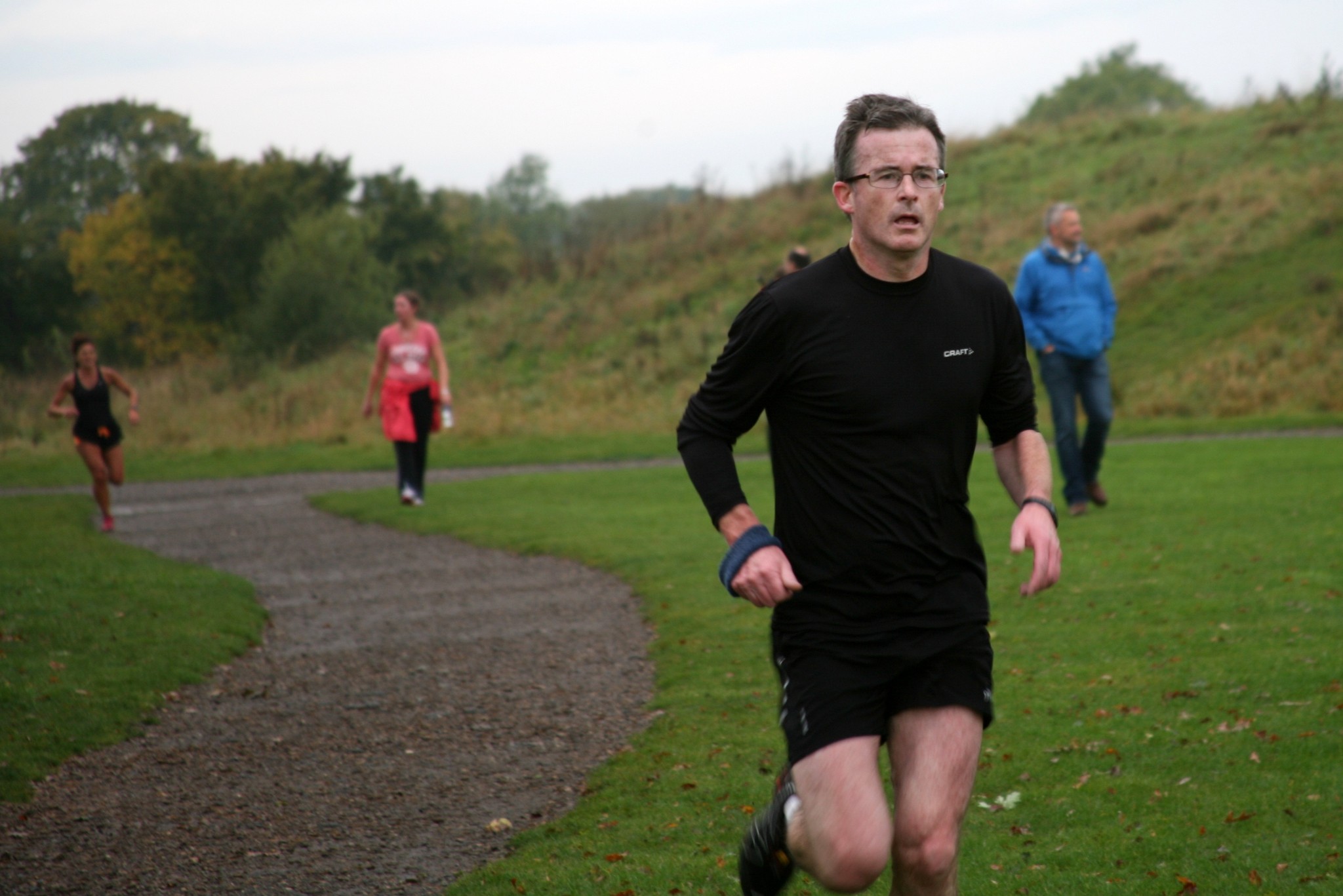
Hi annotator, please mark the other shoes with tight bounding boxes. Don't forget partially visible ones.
[1087,482,1109,506]
[400,488,421,505]
[1069,502,1086,515]
[101,517,114,531]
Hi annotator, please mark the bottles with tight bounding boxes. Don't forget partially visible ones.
[442,403,453,430]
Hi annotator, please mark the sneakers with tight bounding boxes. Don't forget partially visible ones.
[739,773,796,896]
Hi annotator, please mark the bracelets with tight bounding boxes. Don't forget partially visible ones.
[718,524,782,598]
[1021,495,1059,528]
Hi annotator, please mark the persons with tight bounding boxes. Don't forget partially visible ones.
[673,95,1063,895]
[1016,201,1117,518]
[360,291,453,506]
[782,245,811,275]
[46,335,140,535]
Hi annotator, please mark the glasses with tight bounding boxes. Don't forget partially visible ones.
[843,165,950,189]
